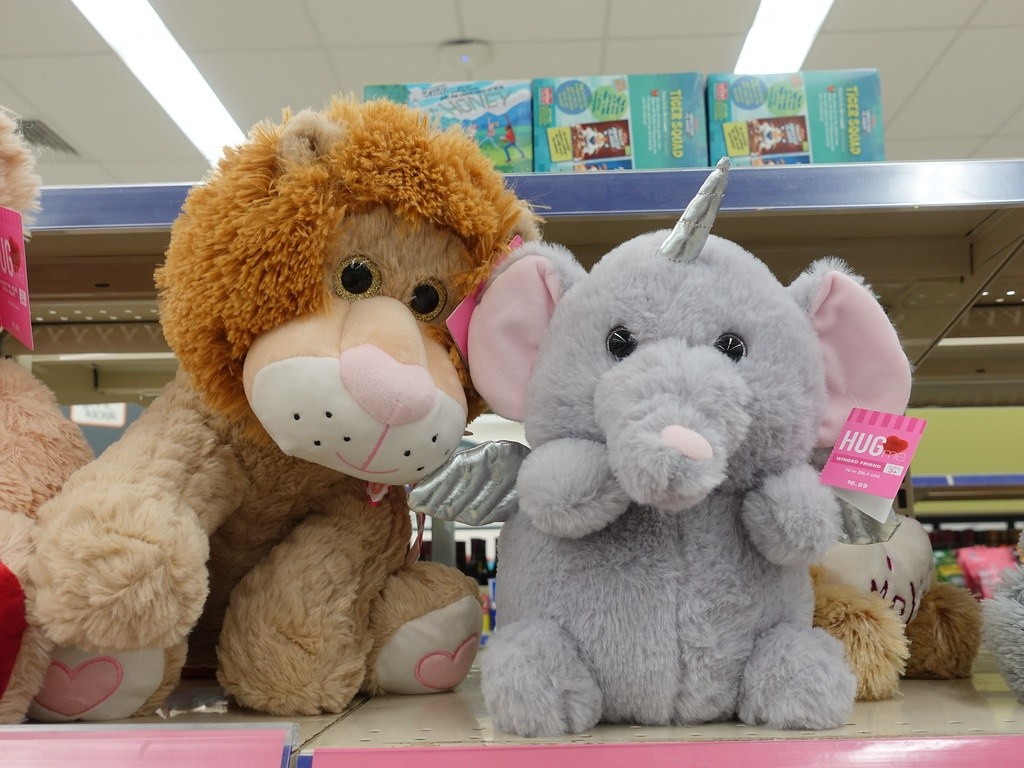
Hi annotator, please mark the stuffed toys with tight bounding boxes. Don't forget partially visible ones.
[810,515,982,701]
[0,106,96,729]
[31,101,544,723]
[467,160,913,734]
[979,530,1024,709]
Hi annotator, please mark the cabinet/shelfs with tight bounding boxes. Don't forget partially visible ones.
[1,160,1024,768]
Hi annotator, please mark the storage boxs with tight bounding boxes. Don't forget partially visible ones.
[533,73,709,172]
[365,82,533,175]
[708,69,887,170]
